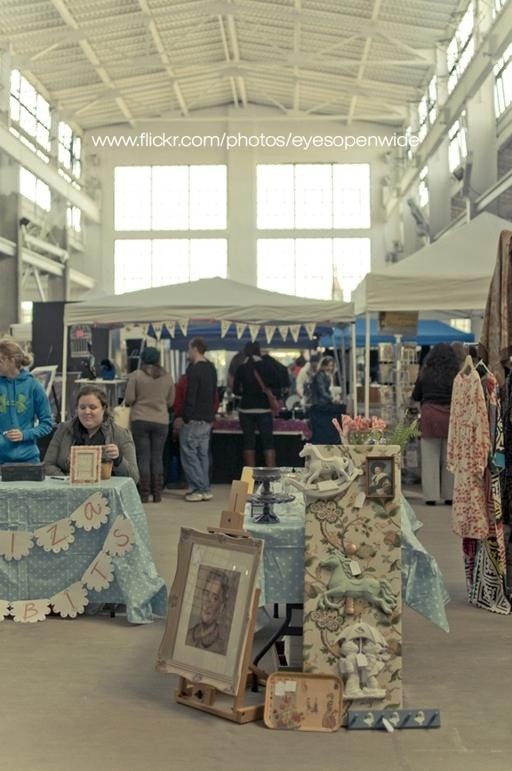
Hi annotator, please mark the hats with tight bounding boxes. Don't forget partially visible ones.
[140,347,160,365]
[371,462,386,474]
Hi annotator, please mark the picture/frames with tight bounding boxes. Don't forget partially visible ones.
[69,446,102,486]
[153,523,265,696]
[365,455,396,500]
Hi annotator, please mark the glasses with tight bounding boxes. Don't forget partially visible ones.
[1,355,17,364]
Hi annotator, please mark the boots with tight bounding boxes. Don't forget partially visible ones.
[153,471,164,502]
[141,472,150,503]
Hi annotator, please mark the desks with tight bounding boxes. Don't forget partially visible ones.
[0,474,170,625]
[74,376,128,406]
[245,463,454,693]
[210,418,312,441]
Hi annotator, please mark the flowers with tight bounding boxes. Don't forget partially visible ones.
[332,408,423,454]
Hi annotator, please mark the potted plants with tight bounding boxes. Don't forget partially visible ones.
[100,458,113,480]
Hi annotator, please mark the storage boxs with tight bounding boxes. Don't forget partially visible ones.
[1,462,46,481]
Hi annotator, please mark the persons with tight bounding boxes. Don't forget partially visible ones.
[227,339,342,467]
[42,386,141,482]
[186,569,231,655]
[125,341,217,506]
[1,340,53,464]
[339,643,385,692]
[410,343,511,506]
[80,358,116,381]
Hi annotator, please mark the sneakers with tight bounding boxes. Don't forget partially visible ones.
[185,491,203,502]
[202,491,213,501]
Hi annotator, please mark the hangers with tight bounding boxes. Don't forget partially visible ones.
[458,342,474,374]
[474,358,491,376]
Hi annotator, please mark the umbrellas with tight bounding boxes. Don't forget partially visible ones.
[332,615,387,654]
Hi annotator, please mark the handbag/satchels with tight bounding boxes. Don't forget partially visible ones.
[253,368,281,411]
[113,399,132,429]
